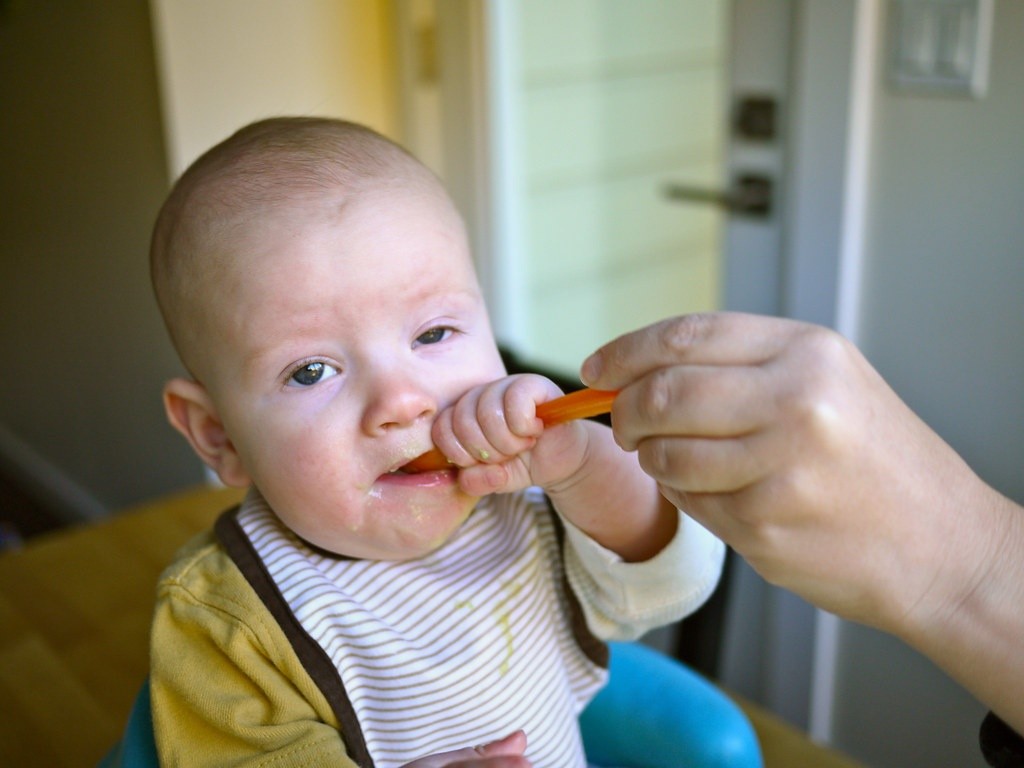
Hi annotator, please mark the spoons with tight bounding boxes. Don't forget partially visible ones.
[395,388,617,473]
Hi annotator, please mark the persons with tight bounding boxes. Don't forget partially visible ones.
[579,310,1024,768]
[149,115,730,768]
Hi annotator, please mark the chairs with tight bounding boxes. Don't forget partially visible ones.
[0,488,855,768]
